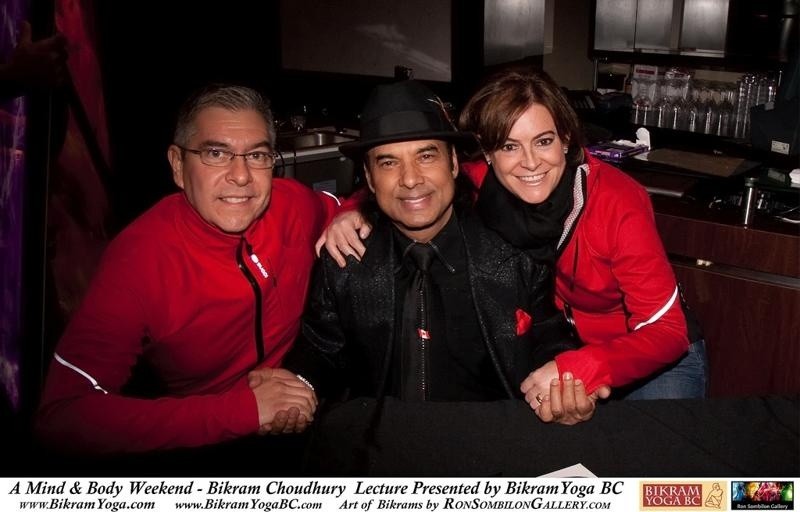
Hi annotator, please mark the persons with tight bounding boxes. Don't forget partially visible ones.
[314,67,709,416]
[248,81,612,437]
[624,83,634,124]
[36,84,343,456]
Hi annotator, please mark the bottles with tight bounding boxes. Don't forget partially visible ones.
[741,175,758,227]
[623,64,777,139]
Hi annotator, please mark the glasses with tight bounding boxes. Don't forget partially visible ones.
[173,142,277,170]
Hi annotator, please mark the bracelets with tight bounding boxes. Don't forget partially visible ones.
[296,374,314,391]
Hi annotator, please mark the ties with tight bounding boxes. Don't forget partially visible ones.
[400,241,437,401]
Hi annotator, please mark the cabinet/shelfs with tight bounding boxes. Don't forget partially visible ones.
[634,0,736,63]
[590,1,640,57]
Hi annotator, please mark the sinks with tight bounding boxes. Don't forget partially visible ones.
[276,130,357,152]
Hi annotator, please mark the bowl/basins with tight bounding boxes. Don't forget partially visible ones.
[292,130,334,148]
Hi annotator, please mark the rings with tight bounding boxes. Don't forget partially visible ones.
[537,394,542,404]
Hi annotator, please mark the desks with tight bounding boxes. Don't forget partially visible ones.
[2,368,800,474]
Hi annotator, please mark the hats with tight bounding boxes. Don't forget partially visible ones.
[339,82,481,164]
